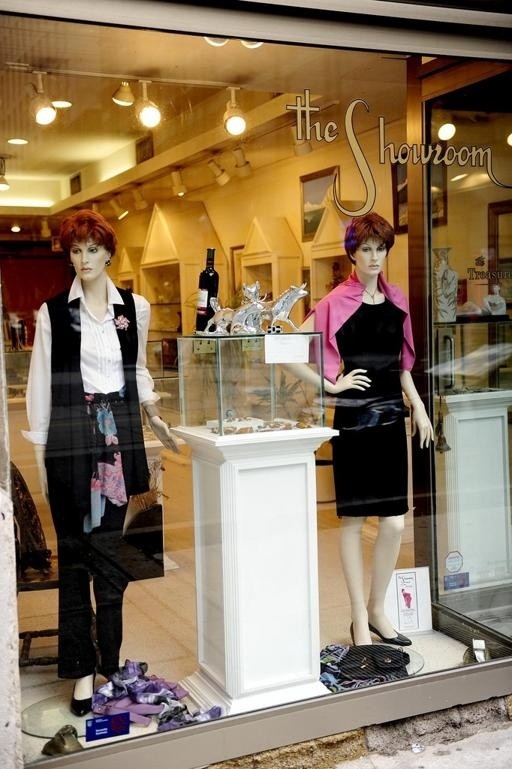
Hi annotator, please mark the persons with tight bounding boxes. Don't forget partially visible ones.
[285,214,439,655]
[26,210,180,716]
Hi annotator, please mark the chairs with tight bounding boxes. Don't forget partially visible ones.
[11,460,105,671]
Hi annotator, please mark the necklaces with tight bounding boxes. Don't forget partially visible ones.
[363,288,378,305]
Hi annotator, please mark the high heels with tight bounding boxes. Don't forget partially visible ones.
[72,669,96,716]
[368,622,412,646]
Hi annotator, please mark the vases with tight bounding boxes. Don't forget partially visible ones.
[431,247,458,323]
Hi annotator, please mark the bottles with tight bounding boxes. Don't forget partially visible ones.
[195,248,219,331]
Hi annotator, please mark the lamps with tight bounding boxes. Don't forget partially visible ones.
[430,111,458,139]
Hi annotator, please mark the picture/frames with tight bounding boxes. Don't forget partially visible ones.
[391,140,448,235]
[229,245,243,295]
[300,165,340,242]
